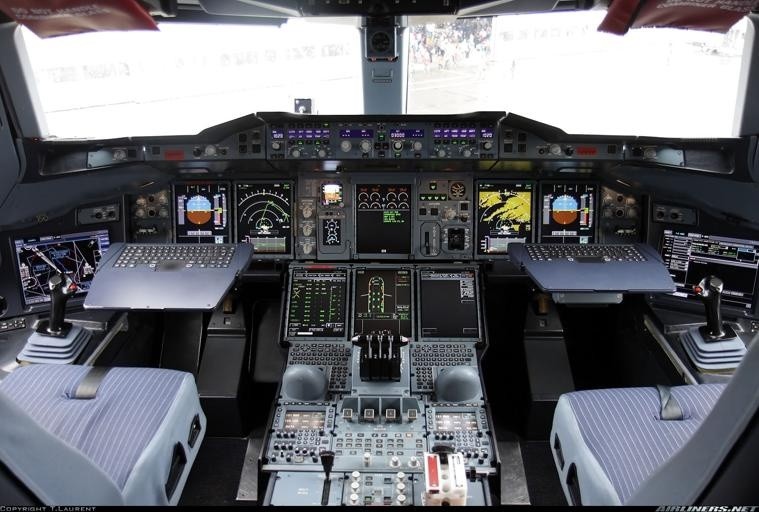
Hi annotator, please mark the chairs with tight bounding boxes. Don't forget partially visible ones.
[1,361,209,508]
[548,334,757,507]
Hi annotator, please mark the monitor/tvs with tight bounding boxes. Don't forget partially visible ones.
[8,224,113,307]
[417,267,482,342]
[652,225,759,310]
[172,180,232,244]
[234,180,293,256]
[351,266,415,342]
[474,179,536,260]
[537,179,599,245]
[283,266,350,340]
[355,182,412,255]
